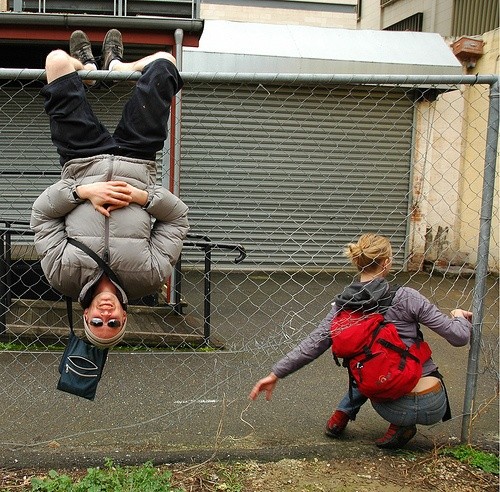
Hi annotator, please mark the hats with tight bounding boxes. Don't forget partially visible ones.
[83,315,128,348]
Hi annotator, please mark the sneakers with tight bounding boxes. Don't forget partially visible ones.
[70,29,99,89]
[101,28,124,89]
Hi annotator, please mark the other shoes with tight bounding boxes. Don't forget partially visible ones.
[325,410,350,436]
[375,422,418,450]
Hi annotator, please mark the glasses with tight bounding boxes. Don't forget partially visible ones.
[88,317,123,328]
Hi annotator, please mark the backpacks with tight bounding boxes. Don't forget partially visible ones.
[330,283,431,400]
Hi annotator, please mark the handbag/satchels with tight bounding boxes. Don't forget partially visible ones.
[55,332,110,403]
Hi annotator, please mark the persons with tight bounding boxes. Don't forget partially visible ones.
[248,233,473,448]
[29,29,190,349]
